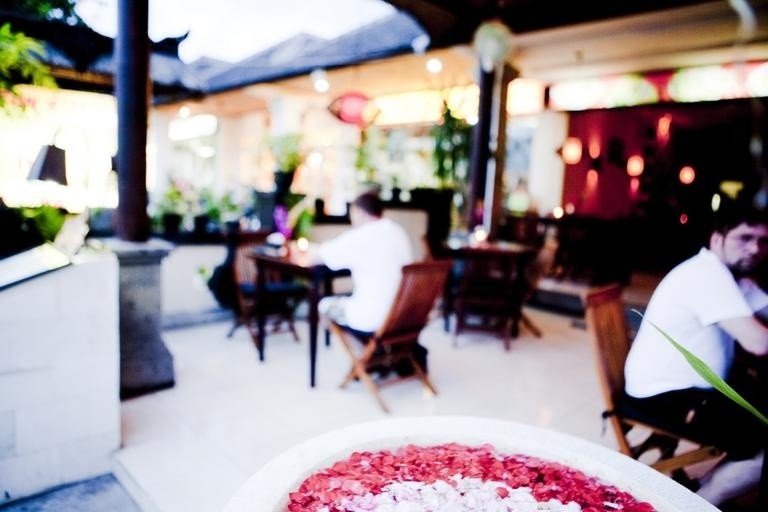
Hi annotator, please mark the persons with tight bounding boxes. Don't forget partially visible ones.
[619,211,768,506]
[296,193,415,379]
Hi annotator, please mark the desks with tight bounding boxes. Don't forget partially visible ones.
[245,247,349,387]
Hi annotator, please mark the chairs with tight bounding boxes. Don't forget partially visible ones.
[429,208,552,352]
[328,255,454,411]
[211,223,304,347]
[575,279,731,489]
